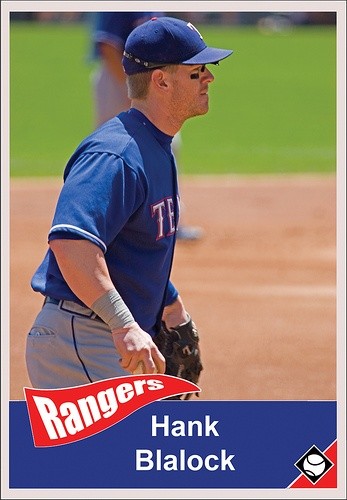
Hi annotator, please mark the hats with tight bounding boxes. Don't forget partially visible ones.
[122,17,233,74]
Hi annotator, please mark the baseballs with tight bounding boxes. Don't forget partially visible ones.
[130,362,159,374]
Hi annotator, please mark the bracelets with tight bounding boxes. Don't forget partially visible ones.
[88,288,136,330]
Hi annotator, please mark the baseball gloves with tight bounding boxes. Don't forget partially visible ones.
[154,312,202,400]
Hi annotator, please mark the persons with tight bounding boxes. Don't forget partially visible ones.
[25,16,235,400]
[85,10,205,243]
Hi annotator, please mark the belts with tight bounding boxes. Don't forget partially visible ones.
[34,292,107,321]
